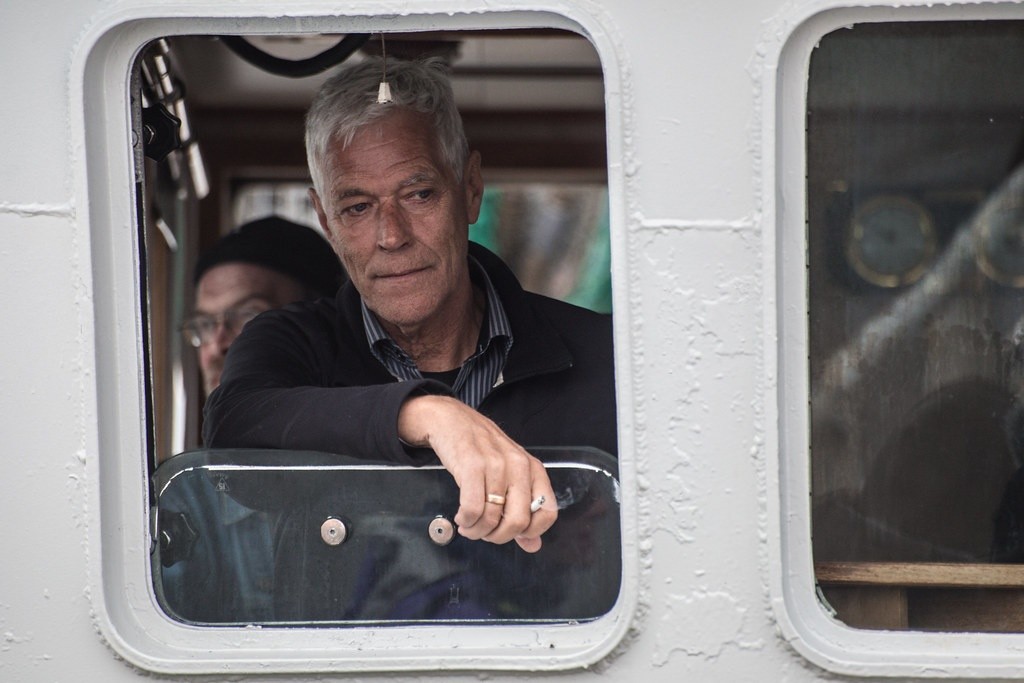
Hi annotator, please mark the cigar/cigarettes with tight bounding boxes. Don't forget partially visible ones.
[530,496,546,513]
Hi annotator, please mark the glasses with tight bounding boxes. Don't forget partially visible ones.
[178,306,257,348]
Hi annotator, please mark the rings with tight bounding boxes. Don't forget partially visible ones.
[485,493,506,505]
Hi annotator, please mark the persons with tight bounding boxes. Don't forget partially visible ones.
[201,56,620,627]
[148,215,359,625]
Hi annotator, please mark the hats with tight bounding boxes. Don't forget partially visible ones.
[192,215,346,296]
